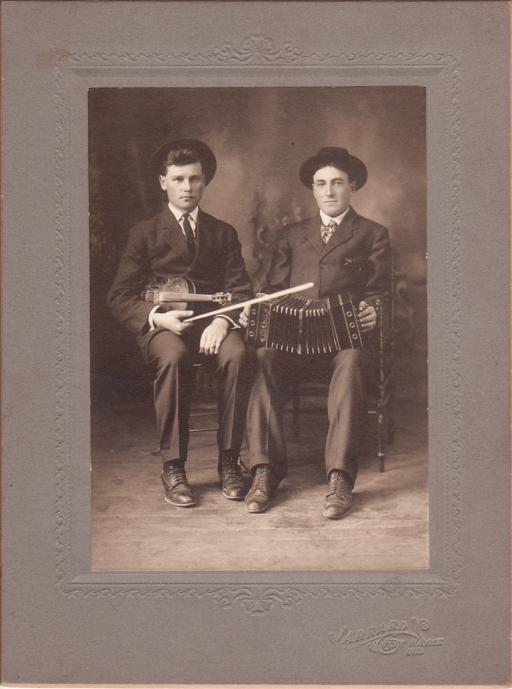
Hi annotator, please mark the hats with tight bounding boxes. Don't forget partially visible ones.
[146,136,218,187]
[298,145,369,190]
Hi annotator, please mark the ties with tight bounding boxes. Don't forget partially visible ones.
[182,214,195,248]
[321,218,339,244]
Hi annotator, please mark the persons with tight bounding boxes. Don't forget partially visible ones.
[238,147,392,519]
[106,139,254,506]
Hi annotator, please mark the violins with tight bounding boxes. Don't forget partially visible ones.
[140,270,233,312]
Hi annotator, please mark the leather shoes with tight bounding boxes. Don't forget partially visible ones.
[217,450,246,499]
[322,471,354,519]
[160,465,197,506]
[244,466,287,514]
